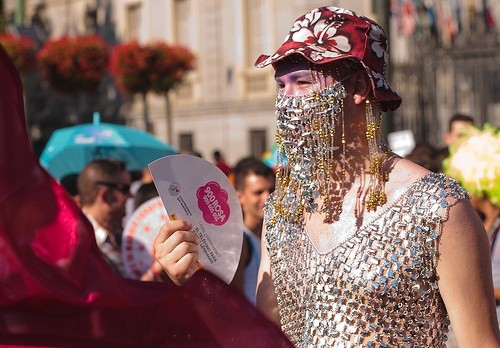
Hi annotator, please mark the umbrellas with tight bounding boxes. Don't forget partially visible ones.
[37,111,179,183]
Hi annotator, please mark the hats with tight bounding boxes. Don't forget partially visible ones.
[255,6,401,111]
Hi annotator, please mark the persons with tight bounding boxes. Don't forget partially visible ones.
[154,6,500,348]
[60,150,277,306]
[403,114,500,328]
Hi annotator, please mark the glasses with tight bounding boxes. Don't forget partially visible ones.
[96,181,128,190]
